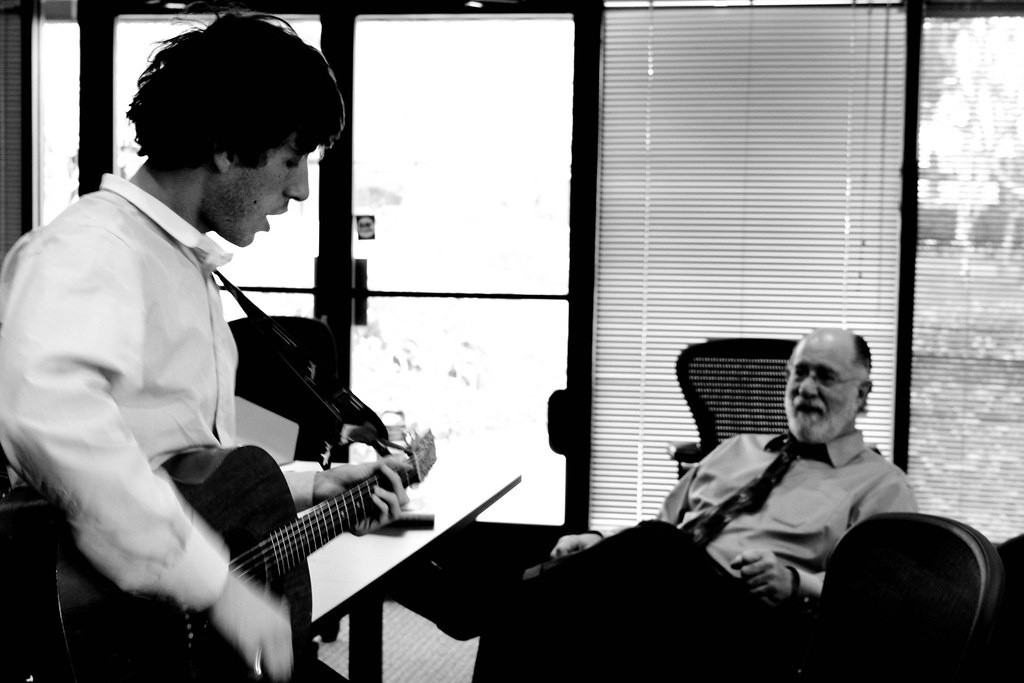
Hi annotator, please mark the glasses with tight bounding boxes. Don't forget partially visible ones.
[785,360,861,387]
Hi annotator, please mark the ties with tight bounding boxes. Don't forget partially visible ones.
[680,440,798,547]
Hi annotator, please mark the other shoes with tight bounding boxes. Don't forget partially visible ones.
[385,560,472,640]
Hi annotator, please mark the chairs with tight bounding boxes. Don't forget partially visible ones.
[669,339,799,479]
[801,517,1006,683]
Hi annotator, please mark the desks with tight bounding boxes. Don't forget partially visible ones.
[280,459,522,683]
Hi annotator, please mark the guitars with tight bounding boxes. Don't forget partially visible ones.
[0,428,437,683]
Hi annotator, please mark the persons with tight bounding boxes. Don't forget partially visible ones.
[380,329,917,683]
[0,11,416,683]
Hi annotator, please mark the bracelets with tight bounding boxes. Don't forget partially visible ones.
[764,565,801,605]
